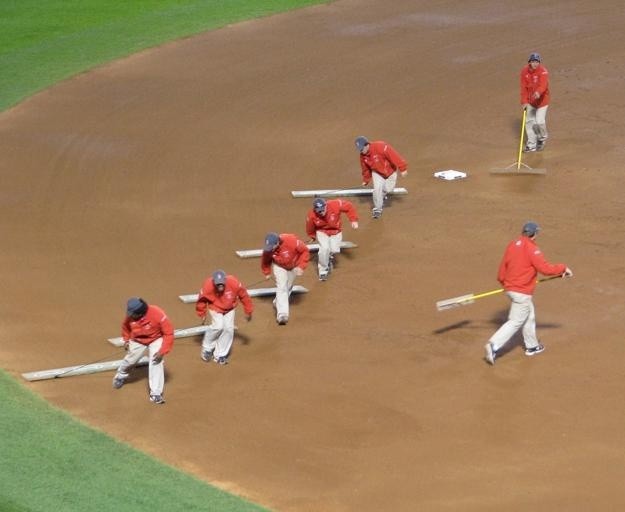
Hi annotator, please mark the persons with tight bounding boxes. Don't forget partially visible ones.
[111,298,175,404]
[305,198,359,281]
[353,135,409,220]
[484,221,572,366]
[519,53,552,154]
[259,233,310,326]
[195,270,253,366]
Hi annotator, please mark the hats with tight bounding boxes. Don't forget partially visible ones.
[522,223,542,233]
[213,270,225,285]
[355,136,368,150]
[126,298,144,317]
[312,199,326,212]
[264,232,278,252]
[528,53,541,63]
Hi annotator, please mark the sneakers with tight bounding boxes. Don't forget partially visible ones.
[201,351,211,361]
[484,343,496,365]
[536,140,546,151]
[319,274,328,281]
[278,316,288,325]
[112,378,125,389]
[372,212,381,218]
[522,146,536,152]
[149,395,165,403]
[525,344,545,356]
[213,357,227,365]
